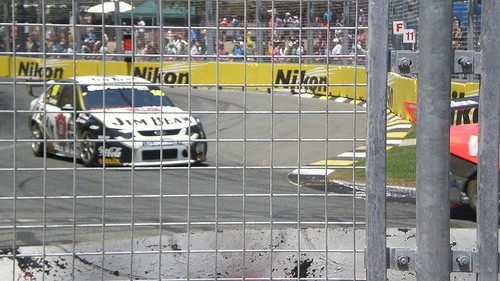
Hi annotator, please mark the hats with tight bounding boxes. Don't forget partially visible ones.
[331,37,340,43]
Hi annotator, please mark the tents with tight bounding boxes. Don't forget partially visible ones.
[85,0,199,24]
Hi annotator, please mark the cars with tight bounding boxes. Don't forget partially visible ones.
[405,92,478,217]
[28,74,208,167]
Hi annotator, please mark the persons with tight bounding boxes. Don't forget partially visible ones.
[0,0,463,64]
